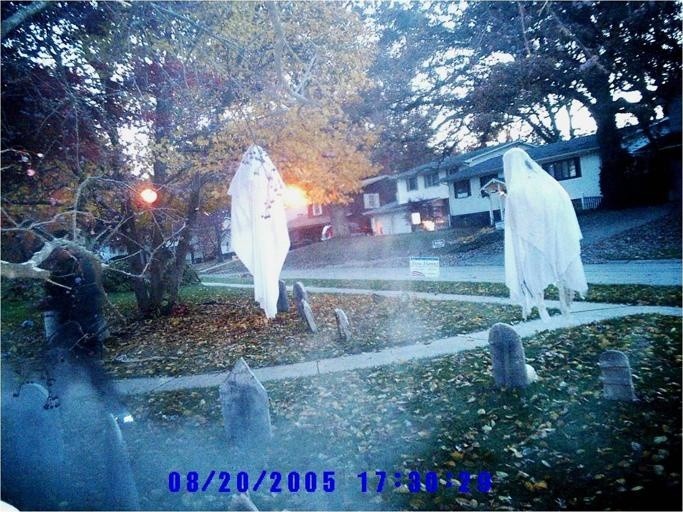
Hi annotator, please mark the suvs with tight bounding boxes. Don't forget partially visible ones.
[320,224,373,241]
